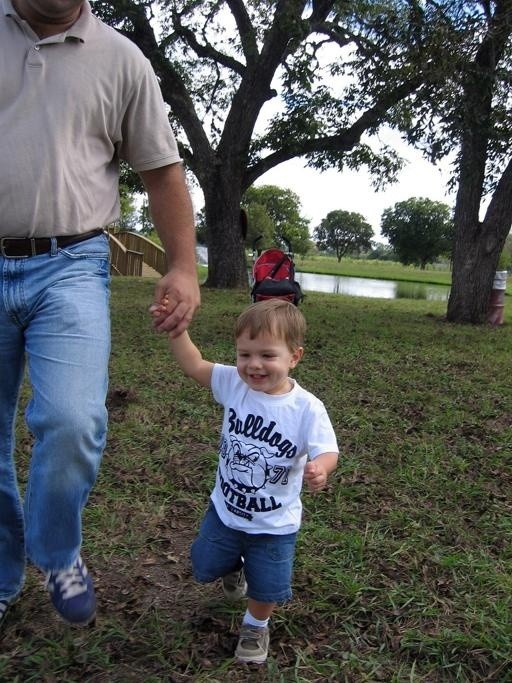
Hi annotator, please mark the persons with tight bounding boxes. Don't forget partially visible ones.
[0,0,201,630]
[151,294,339,664]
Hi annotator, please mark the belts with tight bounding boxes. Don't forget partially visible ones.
[0,228,103,259]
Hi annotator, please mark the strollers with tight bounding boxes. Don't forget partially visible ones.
[252,247,303,307]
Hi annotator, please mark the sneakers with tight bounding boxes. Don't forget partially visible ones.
[235,623,270,664]
[222,568,247,601]
[43,555,96,622]
